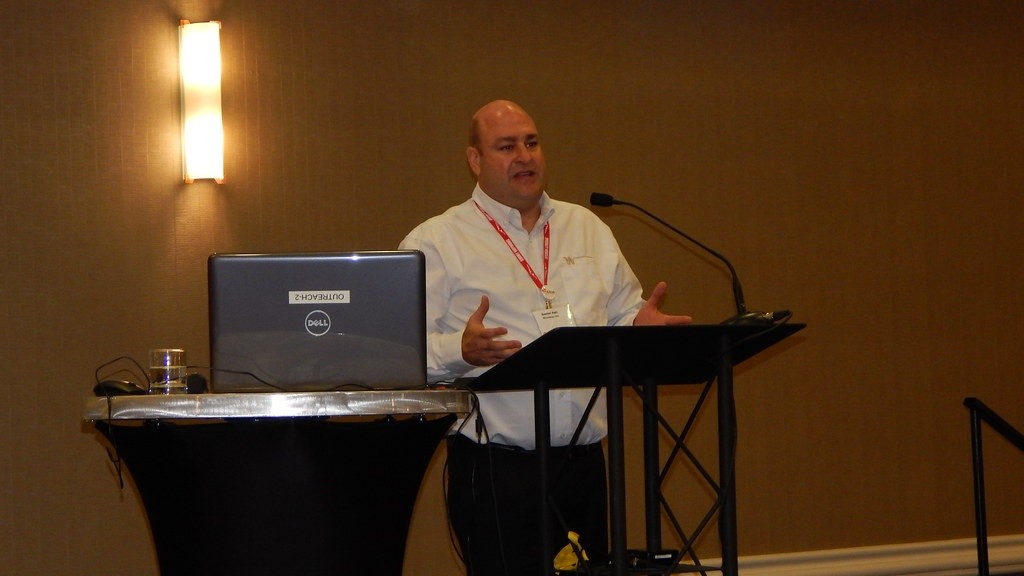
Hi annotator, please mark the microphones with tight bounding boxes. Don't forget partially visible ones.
[182,372,207,394]
[590,191,775,327]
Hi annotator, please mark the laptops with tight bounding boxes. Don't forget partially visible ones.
[208,250,427,390]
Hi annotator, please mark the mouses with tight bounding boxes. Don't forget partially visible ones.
[93,379,148,396]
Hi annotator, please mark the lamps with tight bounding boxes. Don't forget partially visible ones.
[178,19,225,186]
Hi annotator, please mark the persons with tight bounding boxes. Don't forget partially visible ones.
[395,98,695,576]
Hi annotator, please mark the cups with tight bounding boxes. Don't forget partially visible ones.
[145,349,188,395]
